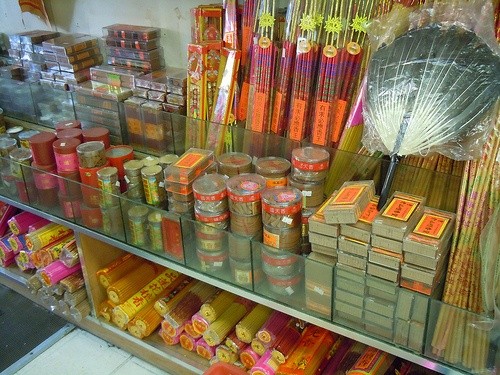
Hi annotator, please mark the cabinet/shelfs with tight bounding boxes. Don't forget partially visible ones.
[0,117,500,375]
